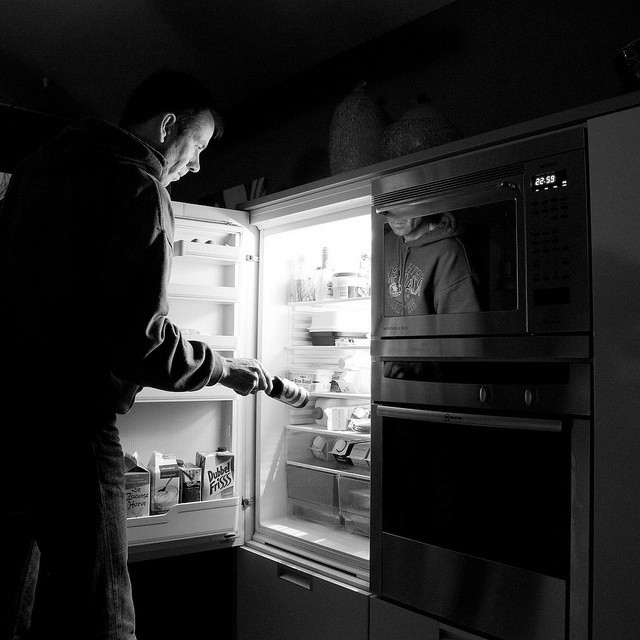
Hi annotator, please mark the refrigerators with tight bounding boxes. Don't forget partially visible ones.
[116,167,370,640]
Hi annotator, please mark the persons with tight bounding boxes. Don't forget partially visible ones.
[0,83,273,640]
[383,205,490,387]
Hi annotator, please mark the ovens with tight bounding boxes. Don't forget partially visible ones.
[370,92,640,640]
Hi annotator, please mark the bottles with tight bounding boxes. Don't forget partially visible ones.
[265,375,311,409]
